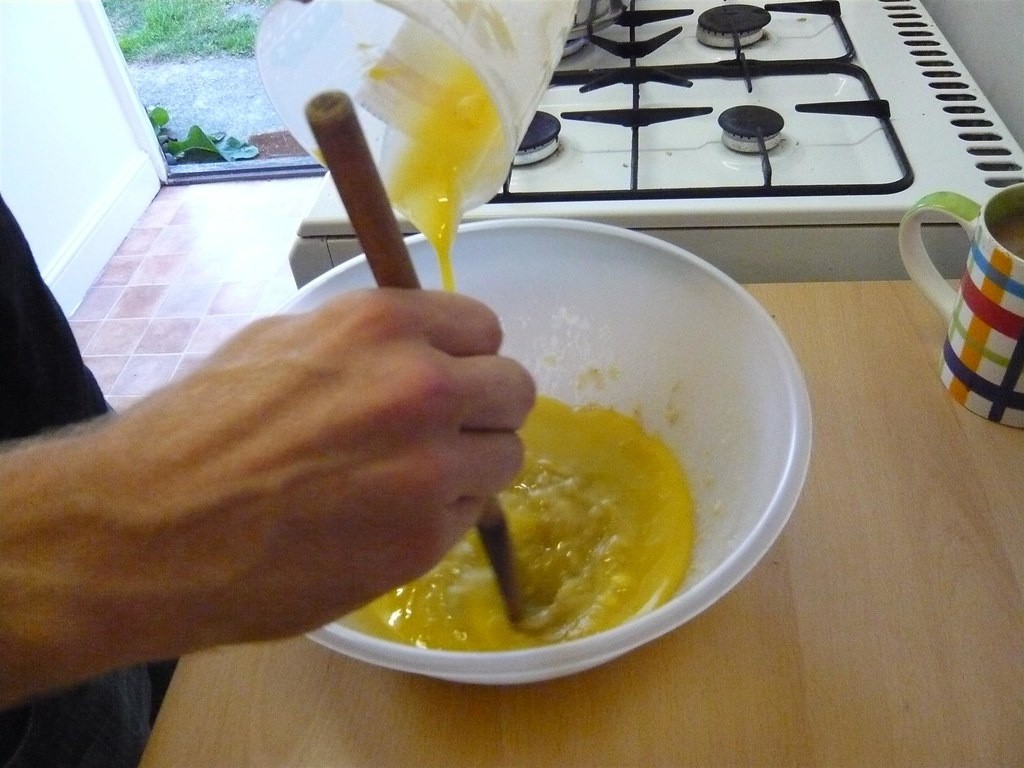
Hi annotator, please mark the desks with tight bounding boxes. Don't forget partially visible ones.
[135,275,1024,767]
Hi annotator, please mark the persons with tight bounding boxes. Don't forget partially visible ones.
[0,198,536,768]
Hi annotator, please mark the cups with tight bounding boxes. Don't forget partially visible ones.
[256,0,576,246]
[896,184,1024,430]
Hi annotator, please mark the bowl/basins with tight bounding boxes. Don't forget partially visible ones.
[275,219,812,685]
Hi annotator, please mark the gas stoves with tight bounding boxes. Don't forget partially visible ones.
[288,2,1024,291]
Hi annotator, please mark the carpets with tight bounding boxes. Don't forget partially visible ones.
[248,130,309,159]
